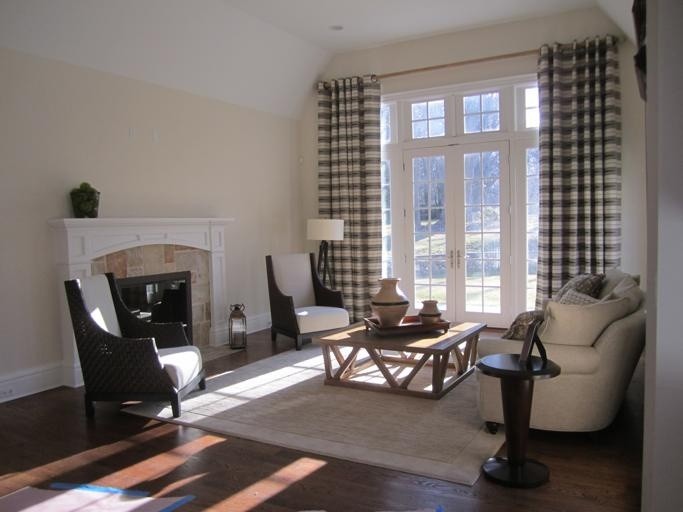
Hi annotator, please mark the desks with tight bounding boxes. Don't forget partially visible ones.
[477,354,562,491]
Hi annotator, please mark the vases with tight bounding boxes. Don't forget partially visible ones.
[371,276,409,327]
[419,299,442,323]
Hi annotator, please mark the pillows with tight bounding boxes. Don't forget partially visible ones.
[557,290,598,304]
[549,273,603,298]
[499,310,544,340]
[599,266,623,298]
[538,298,629,347]
[603,273,644,317]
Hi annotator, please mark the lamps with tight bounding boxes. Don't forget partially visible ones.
[227,303,248,349]
[305,218,345,290]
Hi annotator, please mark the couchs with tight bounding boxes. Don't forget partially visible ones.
[475,270,644,438]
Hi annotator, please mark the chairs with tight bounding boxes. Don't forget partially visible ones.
[264,252,351,352]
[64,270,204,418]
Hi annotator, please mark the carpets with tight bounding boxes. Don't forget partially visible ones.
[118,330,511,491]
[0,475,198,512]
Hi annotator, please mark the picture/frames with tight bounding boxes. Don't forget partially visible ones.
[517,318,551,363]
